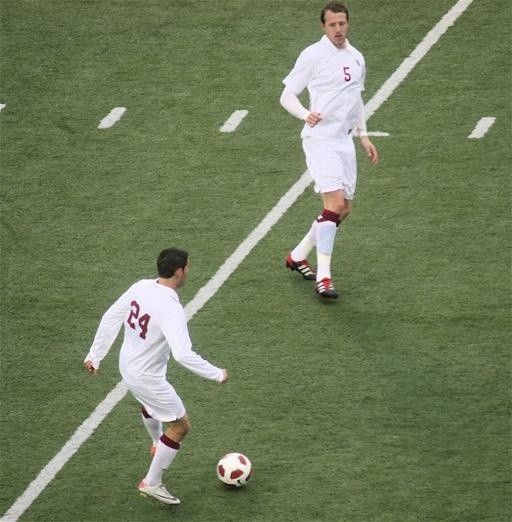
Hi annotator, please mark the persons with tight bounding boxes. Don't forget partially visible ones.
[277,3,379,300]
[84,249,228,504]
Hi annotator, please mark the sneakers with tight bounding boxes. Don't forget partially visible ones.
[136,478,183,507]
[313,276,343,301]
[284,247,318,282]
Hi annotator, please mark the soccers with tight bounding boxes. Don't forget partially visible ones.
[217,453,253,490]
